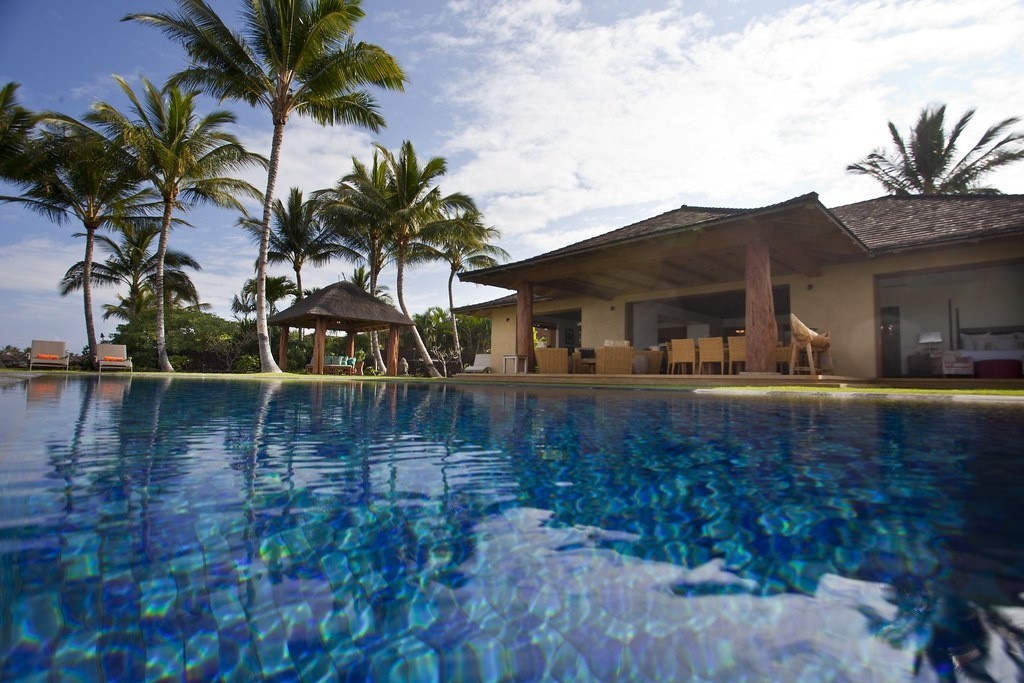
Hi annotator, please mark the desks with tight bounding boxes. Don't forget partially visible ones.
[305,364,352,375]
[696,347,818,375]
[502,355,528,374]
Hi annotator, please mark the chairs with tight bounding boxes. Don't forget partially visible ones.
[92,343,133,373]
[595,346,632,374]
[665,342,672,374]
[634,351,663,373]
[27,340,69,371]
[670,338,696,375]
[572,347,597,373]
[697,337,724,375]
[535,348,568,374]
[463,354,491,373]
[727,336,746,374]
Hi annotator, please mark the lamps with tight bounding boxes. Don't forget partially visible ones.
[917,331,943,357]
